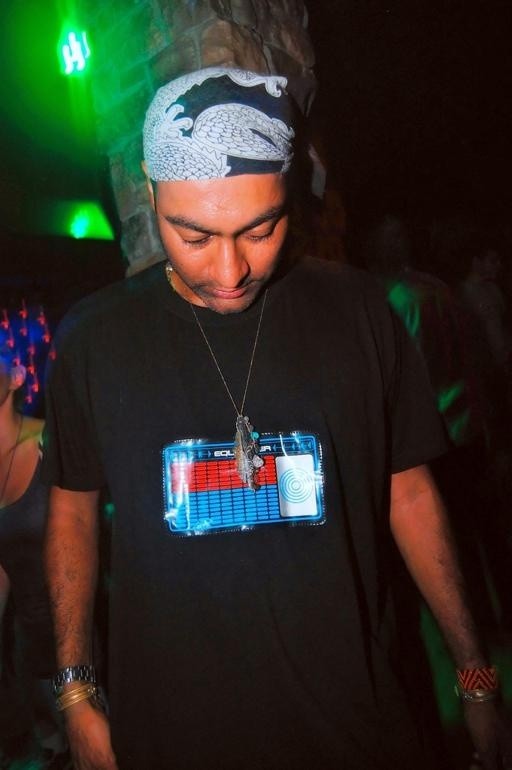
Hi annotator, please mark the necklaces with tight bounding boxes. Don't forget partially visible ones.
[189,289,266,491]
[2,414,22,501]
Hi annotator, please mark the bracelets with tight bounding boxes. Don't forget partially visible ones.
[455,664,498,690]
[461,693,498,703]
[55,682,96,712]
[48,664,98,694]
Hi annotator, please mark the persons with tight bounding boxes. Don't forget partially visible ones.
[1,336,107,770]
[39,65,508,769]
